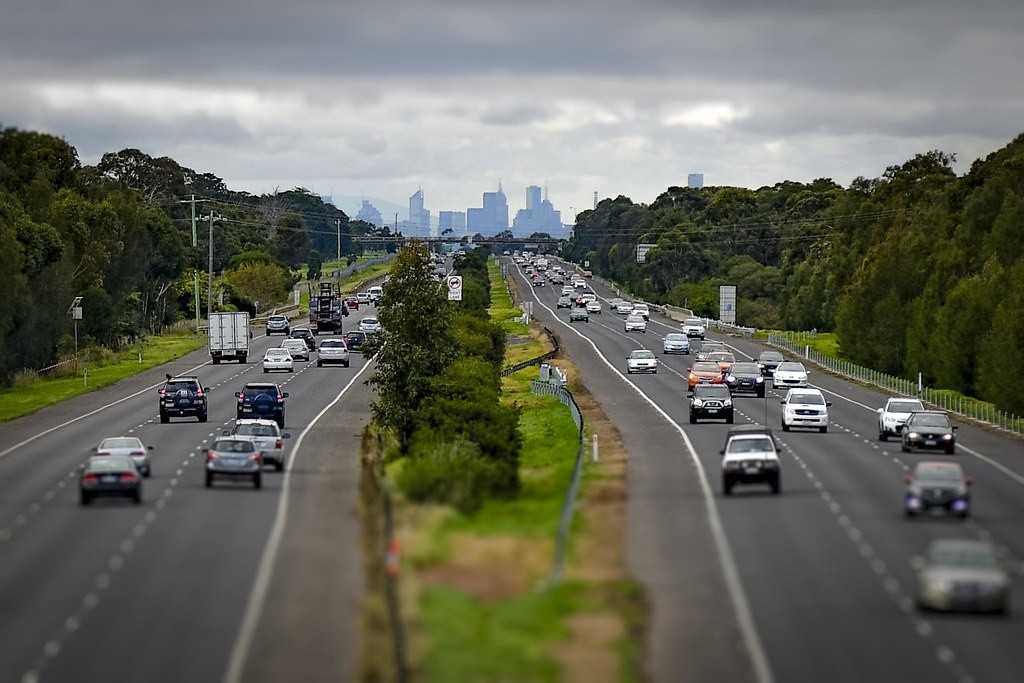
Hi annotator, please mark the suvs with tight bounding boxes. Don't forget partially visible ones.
[235,382,289,428]
[158,374,210,423]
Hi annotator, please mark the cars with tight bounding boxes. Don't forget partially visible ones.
[626,350,659,374]
[662,319,706,355]
[90,436,154,477]
[770,361,810,388]
[222,418,291,470]
[780,388,832,434]
[877,398,925,441]
[902,410,959,454]
[202,435,267,490]
[513,252,650,333]
[263,251,466,373]
[904,462,973,519]
[686,341,765,424]
[910,540,1006,613]
[753,351,789,377]
[78,456,142,503]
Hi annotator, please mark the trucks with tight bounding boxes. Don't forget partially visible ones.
[207,312,253,365]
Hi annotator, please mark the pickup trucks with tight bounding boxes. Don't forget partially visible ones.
[719,424,782,493]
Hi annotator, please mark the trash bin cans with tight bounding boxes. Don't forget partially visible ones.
[539,363,550,383]
